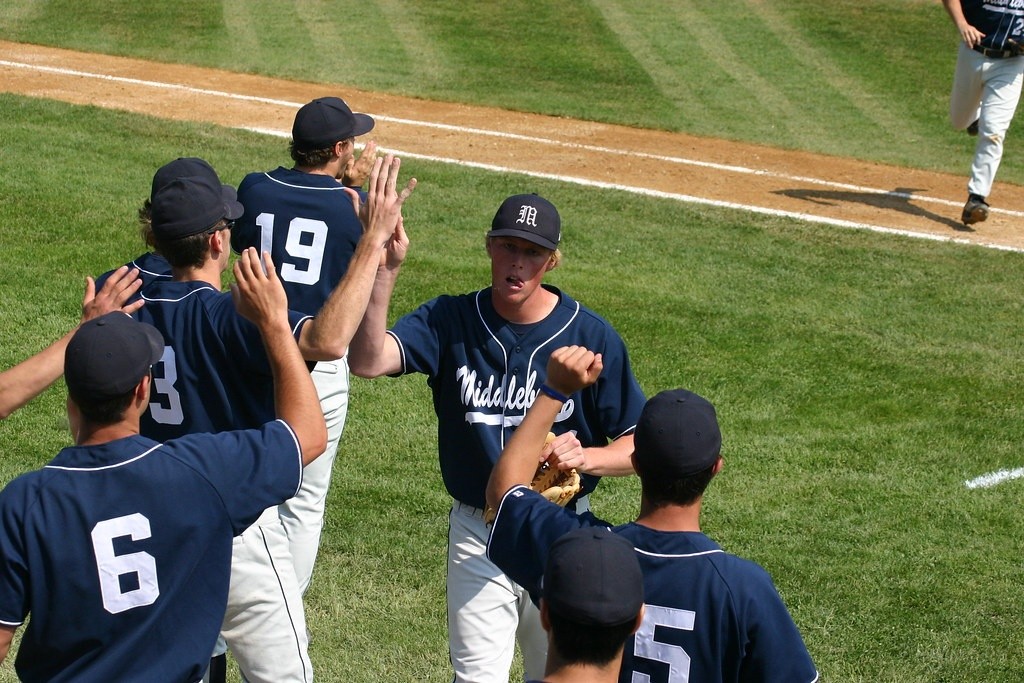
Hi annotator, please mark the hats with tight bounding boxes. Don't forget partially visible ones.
[64,310,165,399]
[539,526,646,625]
[151,157,238,205]
[635,388,722,478]
[151,175,244,242]
[292,97,375,148]
[488,193,562,251]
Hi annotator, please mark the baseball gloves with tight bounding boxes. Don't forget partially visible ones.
[483,432,581,528]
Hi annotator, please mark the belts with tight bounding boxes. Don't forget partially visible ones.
[973,44,1023,58]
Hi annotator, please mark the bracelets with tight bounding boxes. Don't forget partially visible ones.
[539,383,568,403]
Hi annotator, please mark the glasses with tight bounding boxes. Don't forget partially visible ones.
[207,219,236,234]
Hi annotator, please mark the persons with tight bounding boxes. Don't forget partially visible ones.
[0,97,417,683]
[0,247,327,683]
[485,345,819,683]
[517,527,644,683]
[348,193,648,683]
[944,0,1024,224]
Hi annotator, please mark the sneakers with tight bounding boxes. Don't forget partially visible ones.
[961,194,989,224]
[966,119,979,137]
